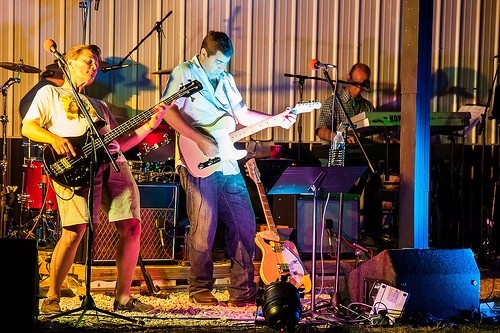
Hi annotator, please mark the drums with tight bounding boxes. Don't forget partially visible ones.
[135,117,175,164]
[20,156,59,215]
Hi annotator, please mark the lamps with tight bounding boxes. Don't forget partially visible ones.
[253,280,304,333]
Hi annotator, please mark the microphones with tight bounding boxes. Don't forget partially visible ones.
[307,59,335,71]
[43,39,66,65]
[101,63,131,72]
[363,79,371,93]
[325,218,333,240]
[155,217,165,247]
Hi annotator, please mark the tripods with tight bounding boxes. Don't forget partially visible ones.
[46,60,145,332]
[271,164,370,324]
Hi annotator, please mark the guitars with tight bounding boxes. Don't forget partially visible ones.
[243,158,305,288]
[178,100,321,178]
[40,78,204,190]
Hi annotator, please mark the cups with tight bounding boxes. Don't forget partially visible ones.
[269,145,282,159]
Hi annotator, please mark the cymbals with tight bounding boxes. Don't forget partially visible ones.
[150,67,173,75]
[45,63,64,73]
[0,61,41,75]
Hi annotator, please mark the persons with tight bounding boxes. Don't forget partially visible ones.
[313,63,388,246]
[163,29,297,306]
[22,44,153,315]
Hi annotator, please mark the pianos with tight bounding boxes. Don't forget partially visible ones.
[311,109,471,248]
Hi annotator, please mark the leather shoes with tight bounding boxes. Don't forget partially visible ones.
[230,292,261,306]
[189,291,219,305]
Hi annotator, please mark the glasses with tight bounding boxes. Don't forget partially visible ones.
[76,43,101,55]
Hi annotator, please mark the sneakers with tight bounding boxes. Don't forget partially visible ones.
[113,296,154,313]
[41,296,61,314]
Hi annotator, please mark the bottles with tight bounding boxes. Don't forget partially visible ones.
[331,131,345,167]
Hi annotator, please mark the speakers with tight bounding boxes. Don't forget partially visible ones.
[72,180,179,265]
[348,248,481,326]
[292,194,361,260]
[0,237,39,333]
